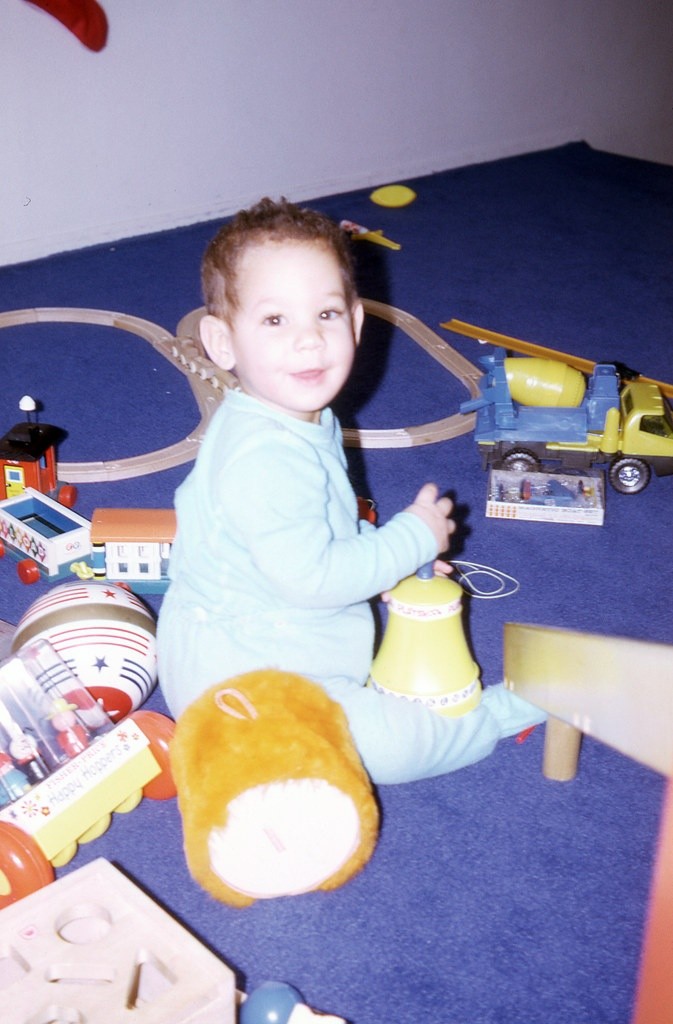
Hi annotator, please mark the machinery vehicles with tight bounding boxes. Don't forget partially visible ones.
[459,346,673,495]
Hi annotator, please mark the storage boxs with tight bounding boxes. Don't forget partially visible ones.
[0,856,239,1024]
[485,469,606,526]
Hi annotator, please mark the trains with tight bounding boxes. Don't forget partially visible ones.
[0,395,376,597]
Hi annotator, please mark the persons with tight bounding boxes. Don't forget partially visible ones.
[157,195,548,785]
